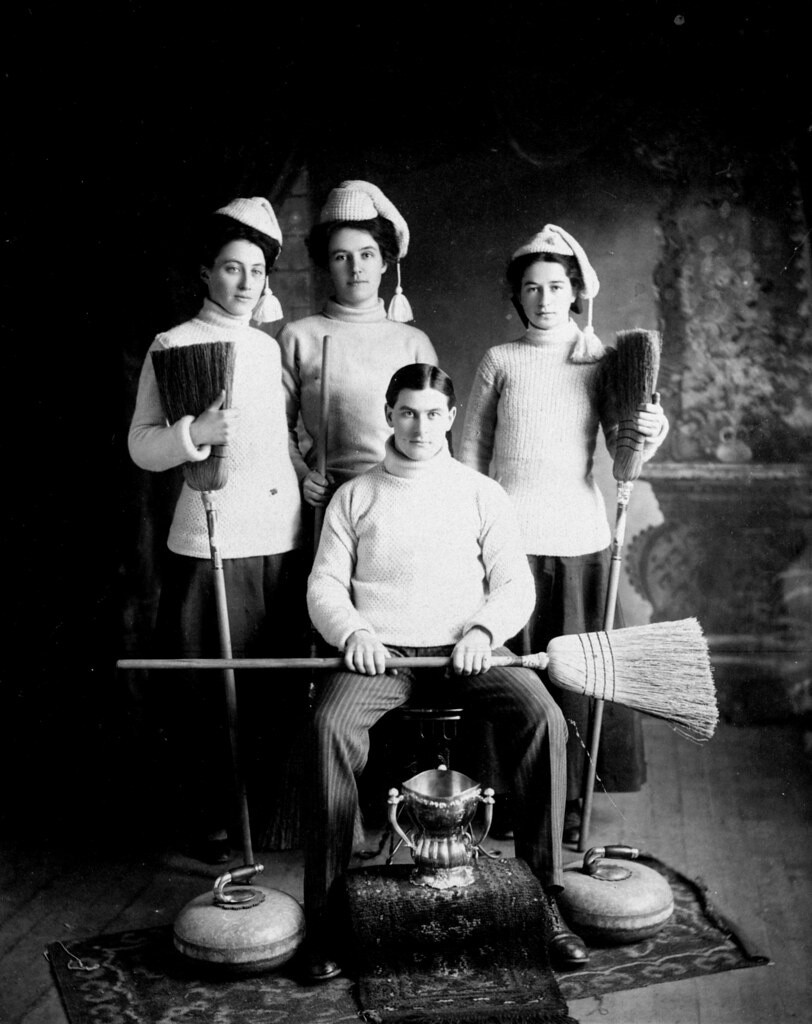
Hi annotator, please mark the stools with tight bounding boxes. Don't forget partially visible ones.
[371,704,488,796]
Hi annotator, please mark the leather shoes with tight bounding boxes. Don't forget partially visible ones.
[535,893,589,963]
[291,913,344,978]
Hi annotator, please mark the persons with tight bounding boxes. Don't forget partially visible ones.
[460,223,668,848]
[300,363,592,980]
[279,179,440,504]
[128,198,300,864]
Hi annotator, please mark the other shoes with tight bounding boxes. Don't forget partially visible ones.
[209,836,232,861]
[563,798,581,843]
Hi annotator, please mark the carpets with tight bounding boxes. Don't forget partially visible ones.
[348,858,579,1024]
[45,856,771,1024]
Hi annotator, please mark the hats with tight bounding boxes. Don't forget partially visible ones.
[511,223,606,364]
[214,197,284,324]
[320,180,414,323]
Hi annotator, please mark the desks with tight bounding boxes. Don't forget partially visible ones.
[637,462,812,668]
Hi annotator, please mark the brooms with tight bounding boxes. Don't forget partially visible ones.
[261,334,368,858]
[576,326,661,854]
[115,615,721,747]
[148,339,258,886]
